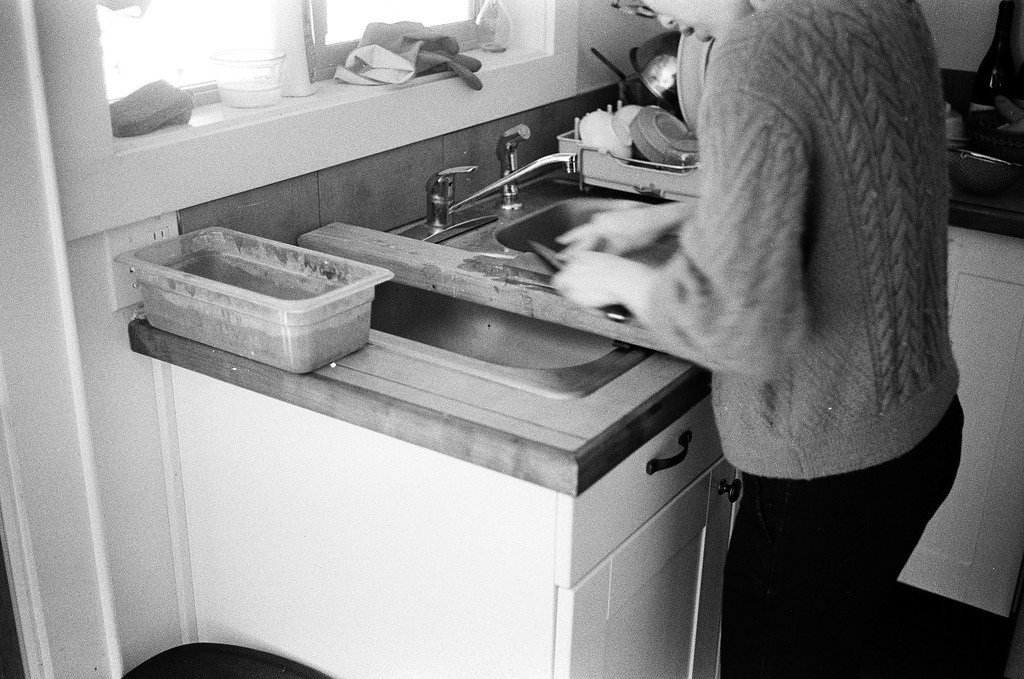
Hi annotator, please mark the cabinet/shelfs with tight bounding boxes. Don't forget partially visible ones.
[170,365,743,679]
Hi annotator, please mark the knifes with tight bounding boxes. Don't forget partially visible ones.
[527,239,632,323]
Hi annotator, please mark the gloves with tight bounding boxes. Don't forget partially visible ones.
[334,20,483,90]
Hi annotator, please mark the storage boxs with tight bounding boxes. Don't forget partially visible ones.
[114,224,395,374]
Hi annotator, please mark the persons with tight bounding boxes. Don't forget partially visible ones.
[546,0,965,678]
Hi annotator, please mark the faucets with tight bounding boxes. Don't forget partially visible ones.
[427,152,578,228]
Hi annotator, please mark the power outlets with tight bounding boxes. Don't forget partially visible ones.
[103,211,179,311]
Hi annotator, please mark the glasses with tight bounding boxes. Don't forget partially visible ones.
[612,0,658,19]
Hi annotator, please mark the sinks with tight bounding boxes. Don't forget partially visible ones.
[490,170,690,264]
[368,279,654,398]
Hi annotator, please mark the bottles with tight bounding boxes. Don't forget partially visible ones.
[969,0,1018,108]
[474,0,512,52]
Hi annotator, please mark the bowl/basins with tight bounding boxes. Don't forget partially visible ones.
[214,51,286,109]
[630,107,689,166]
[612,105,644,146]
[578,109,632,165]
[639,52,678,104]
